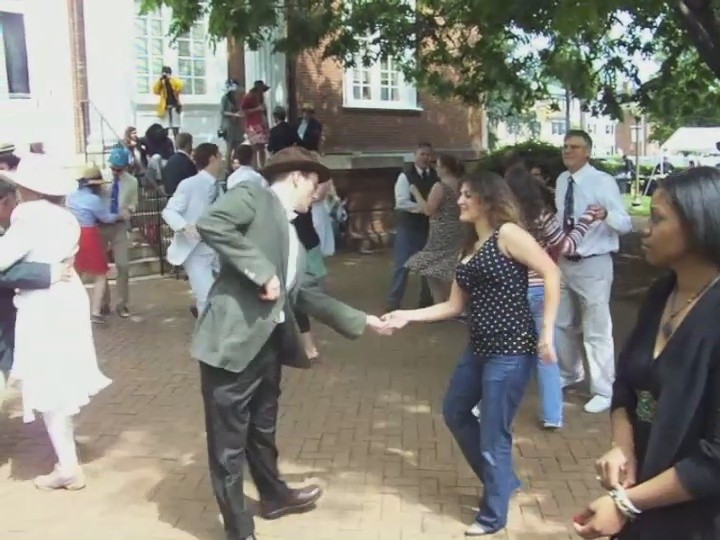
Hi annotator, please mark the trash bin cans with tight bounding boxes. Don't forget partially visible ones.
[616,177,631,193]
[641,177,657,196]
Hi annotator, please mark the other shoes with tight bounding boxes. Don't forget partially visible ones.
[584,392,612,412]
[560,374,585,388]
[92,315,105,323]
[543,420,563,427]
[103,302,111,315]
[465,522,486,535]
[116,304,130,317]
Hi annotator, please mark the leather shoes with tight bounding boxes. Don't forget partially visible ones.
[264,484,321,518]
[244,534,255,540]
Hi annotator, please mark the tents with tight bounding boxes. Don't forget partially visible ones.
[644,127,720,194]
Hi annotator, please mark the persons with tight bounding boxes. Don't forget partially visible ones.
[0,138,138,490]
[153,66,182,137]
[554,130,631,413]
[504,163,599,427]
[226,145,261,190]
[382,169,561,536]
[190,147,392,540]
[571,167,720,540]
[406,153,470,320]
[161,144,224,319]
[656,156,674,174]
[291,151,337,361]
[382,143,439,309]
[125,123,197,202]
[623,155,635,177]
[220,79,321,166]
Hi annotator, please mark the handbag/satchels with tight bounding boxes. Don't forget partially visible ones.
[0,263,52,289]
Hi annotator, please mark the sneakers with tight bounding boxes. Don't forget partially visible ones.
[34,461,85,491]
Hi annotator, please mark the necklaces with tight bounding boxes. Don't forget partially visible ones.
[662,274,720,336]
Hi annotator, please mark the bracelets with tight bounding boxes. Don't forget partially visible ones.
[611,489,641,522]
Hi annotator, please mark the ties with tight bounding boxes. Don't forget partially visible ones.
[110,174,119,213]
[423,168,428,180]
[563,176,575,236]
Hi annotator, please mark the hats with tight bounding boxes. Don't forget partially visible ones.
[1,153,80,196]
[111,149,128,167]
[301,103,316,113]
[260,147,332,184]
[249,81,269,92]
[77,166,112,190]
